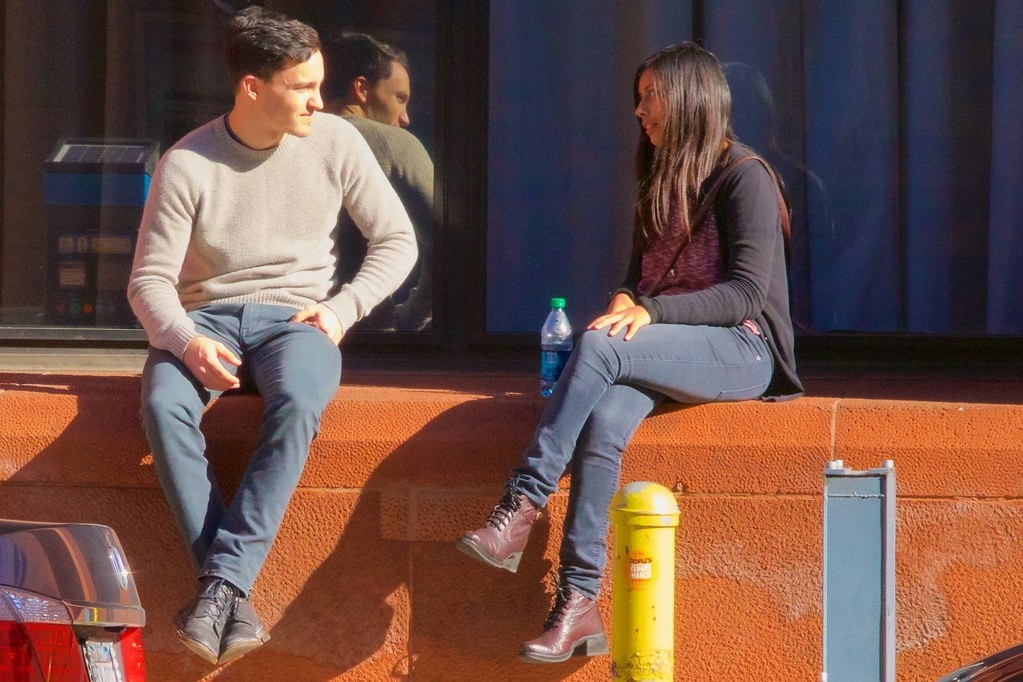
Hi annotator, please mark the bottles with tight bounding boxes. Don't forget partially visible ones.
[540,298,573,398]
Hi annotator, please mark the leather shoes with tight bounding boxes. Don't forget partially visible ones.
[216,596,271,667]
[172,579,238,664]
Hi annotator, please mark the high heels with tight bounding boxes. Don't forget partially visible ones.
[517,571,610,664]
[458,479,541,572]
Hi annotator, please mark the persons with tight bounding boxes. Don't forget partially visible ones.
[456,41,805,661]
[322,28,467,336]
[127,5,420,665]
